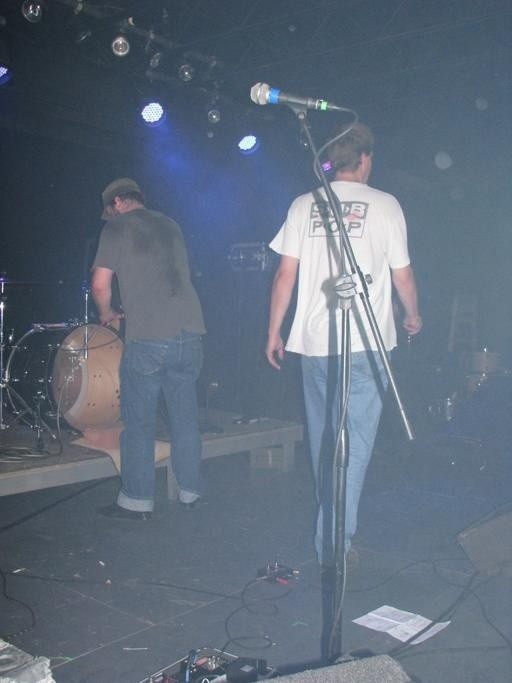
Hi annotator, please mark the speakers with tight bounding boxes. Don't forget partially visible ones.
[457,504,512,576]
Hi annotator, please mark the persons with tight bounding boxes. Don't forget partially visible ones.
[263,118,425,574]
[87,174,208,523]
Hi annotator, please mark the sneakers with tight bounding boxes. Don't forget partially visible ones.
[116,488,154,512]
[177,487,201,510]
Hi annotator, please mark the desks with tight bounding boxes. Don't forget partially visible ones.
[1,405,306,498]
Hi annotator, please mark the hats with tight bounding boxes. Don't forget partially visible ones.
[100,177,144,221]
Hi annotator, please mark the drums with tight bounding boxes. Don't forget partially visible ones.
[424,396,457,426]
[464,350,509,377]
[5,317,124,434]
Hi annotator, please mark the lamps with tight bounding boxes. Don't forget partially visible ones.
[232,130,260,158]
[22,2,44,23]
[137,98,169,130]
[205,105,220,124]
[111,32,130,57]
[178,62,193,84]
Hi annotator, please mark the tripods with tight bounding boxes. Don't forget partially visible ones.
[0,277,70,449]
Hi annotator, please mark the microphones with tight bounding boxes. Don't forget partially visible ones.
[249,78,348,118]
